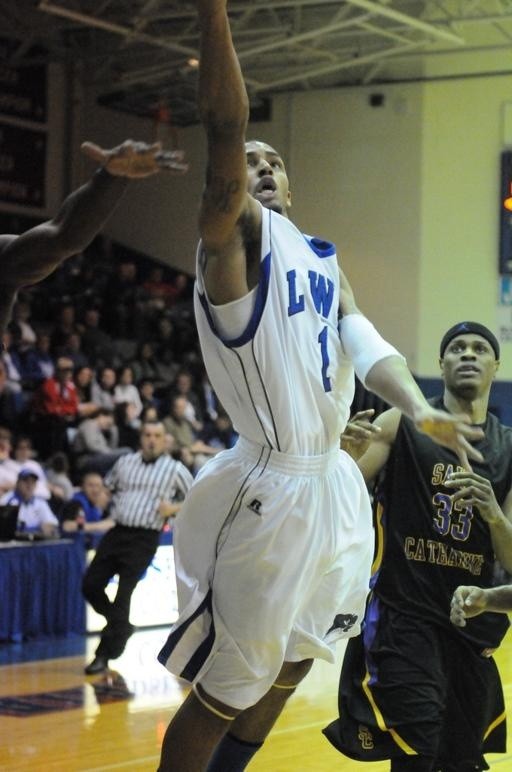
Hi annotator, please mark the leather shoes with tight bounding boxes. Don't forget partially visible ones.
[85,656,107,674]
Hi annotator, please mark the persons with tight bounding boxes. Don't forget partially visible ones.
[321,317,510,769]
[444,580,510,626]
[151,0,489,772]
[78,419,195,674]
[0,216,245,550]
[0,133,194,369]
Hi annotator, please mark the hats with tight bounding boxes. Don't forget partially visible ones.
[19,469,39,483]
